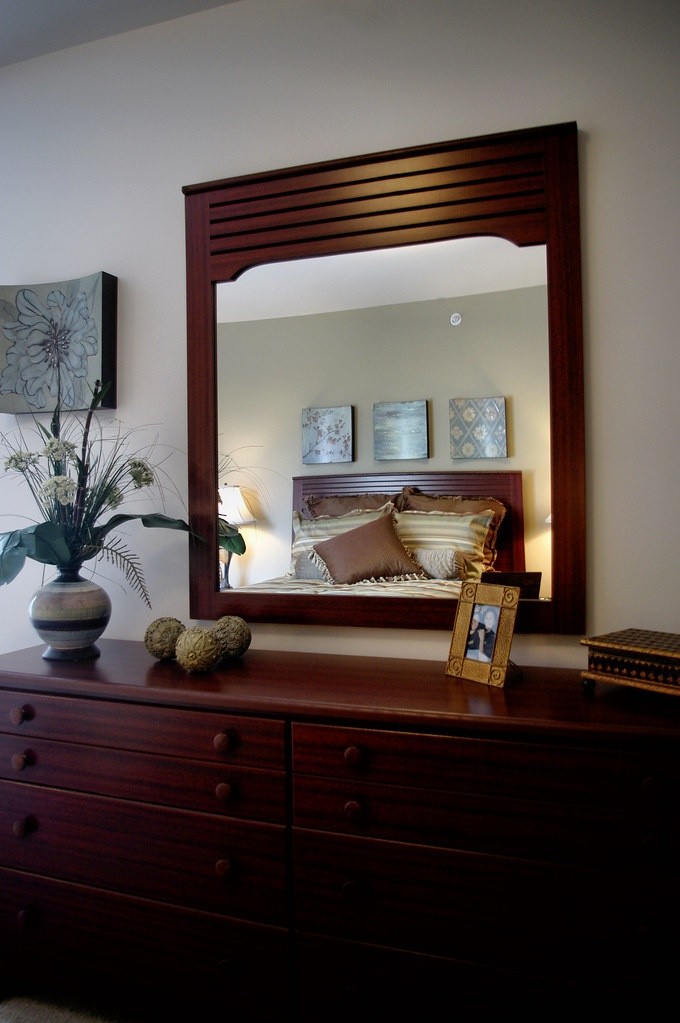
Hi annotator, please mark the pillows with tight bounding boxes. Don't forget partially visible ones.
[291,485,505,586]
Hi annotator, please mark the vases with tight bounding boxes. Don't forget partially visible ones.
[29,566,113,661]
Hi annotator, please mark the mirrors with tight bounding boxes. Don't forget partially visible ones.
[180,119,585,635]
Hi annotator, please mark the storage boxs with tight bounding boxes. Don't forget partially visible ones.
[580,630,680,698]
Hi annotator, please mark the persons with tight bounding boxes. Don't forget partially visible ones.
[465,609,498,663]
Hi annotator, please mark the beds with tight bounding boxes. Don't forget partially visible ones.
[226,471,526,592]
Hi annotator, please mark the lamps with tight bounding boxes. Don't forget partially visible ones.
[217,486,256,590]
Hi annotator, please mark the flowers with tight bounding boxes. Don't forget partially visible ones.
[0,383,202,611]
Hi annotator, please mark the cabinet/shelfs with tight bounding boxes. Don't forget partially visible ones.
[0,635,680,1023]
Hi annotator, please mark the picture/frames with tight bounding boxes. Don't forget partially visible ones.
[445,583,520,688]
